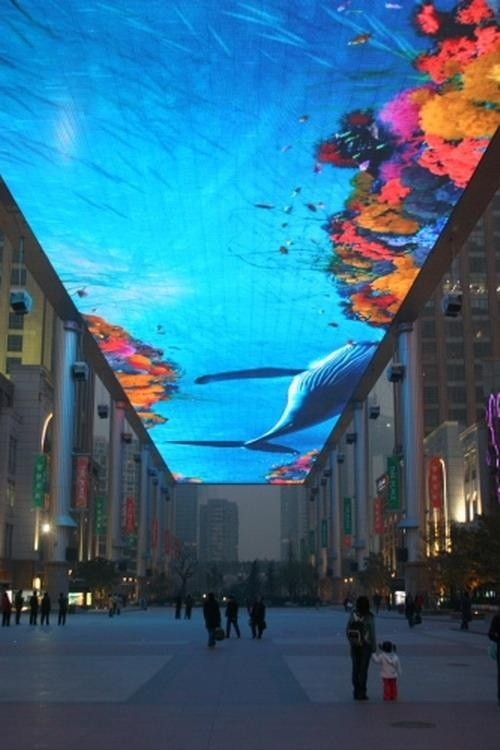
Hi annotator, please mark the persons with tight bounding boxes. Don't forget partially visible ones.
[405,600,417,628]
[0,594,12,627]
[184,595,196,621]
[112,593,118,614]
[247,592,265,640]
[203,593,221,648]
[175,595,182,619]
[15,589,24,625]
[405,592,414,606]
[373,593,381,616]
[460,594,473,631]
[343,598,354,613]
[415,593,425,613]
[57,593,67,626]
[107,593,114,617]
[224,595,240,638]
[116,594,122,615]
[346,595,378,702]
[314,596,321,610]
[29,590,39,625]
[40,593,49,625]
[372,641,402,702]
[488,611,499,707]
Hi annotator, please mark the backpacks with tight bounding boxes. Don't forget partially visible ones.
[347,620,368,648]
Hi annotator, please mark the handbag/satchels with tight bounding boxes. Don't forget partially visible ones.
[214,629,225,641]
[261,622,266,630]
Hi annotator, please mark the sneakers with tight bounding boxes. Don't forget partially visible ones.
[354,695,370,700]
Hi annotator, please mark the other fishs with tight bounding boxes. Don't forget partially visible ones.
[384,2,402,10]
[346,33,371,47]
[328,321,339,328]
[318,308,324,314]
[165,438,303,457]
[336,0,352,12]
[314,161,322,174]
[193,365,307,386]
[279,144,294,154]
[168,346,181,350]
[76,288,89,299]
[242,338,381,448]
[155,323,162,331]
[295,113,308,126]
[252,186,326,256]
